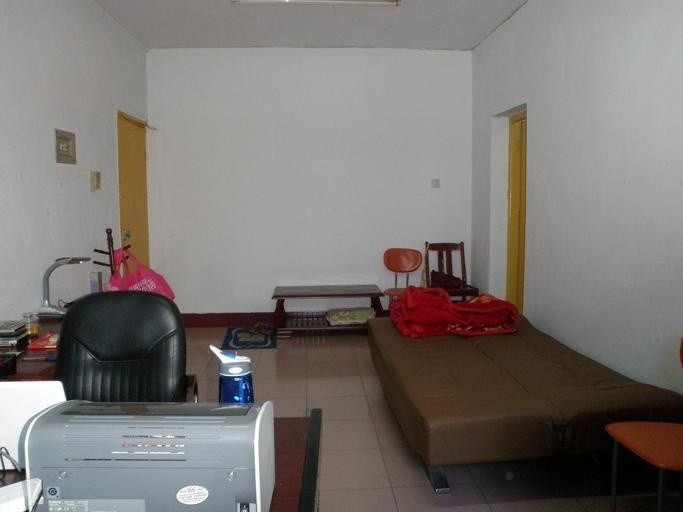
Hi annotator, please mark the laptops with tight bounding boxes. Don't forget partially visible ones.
[0,380,66,471]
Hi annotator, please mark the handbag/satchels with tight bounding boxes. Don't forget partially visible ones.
[104,247,176,301]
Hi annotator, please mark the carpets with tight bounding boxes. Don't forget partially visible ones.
[220,327,277,350]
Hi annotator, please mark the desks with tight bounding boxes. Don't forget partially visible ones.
[0,408,323,512]
[271,285,385,339]
[1,312,61,381]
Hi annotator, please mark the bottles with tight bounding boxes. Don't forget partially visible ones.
[21,312,39,336]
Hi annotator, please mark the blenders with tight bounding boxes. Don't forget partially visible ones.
[209,345,254,403]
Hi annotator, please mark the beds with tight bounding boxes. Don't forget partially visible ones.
[367,314,682,493]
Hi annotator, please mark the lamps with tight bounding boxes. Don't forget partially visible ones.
[230,0,402,7]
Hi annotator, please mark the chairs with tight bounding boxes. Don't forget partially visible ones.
[604,337,683,511]
[54,290,199,403]
[384,247,422,302]
[424,241,480,302]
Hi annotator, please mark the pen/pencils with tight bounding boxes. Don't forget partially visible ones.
[21,355,55,364]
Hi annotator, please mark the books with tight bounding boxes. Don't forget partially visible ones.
[27,329,60,349]
[0,320,27,358]
